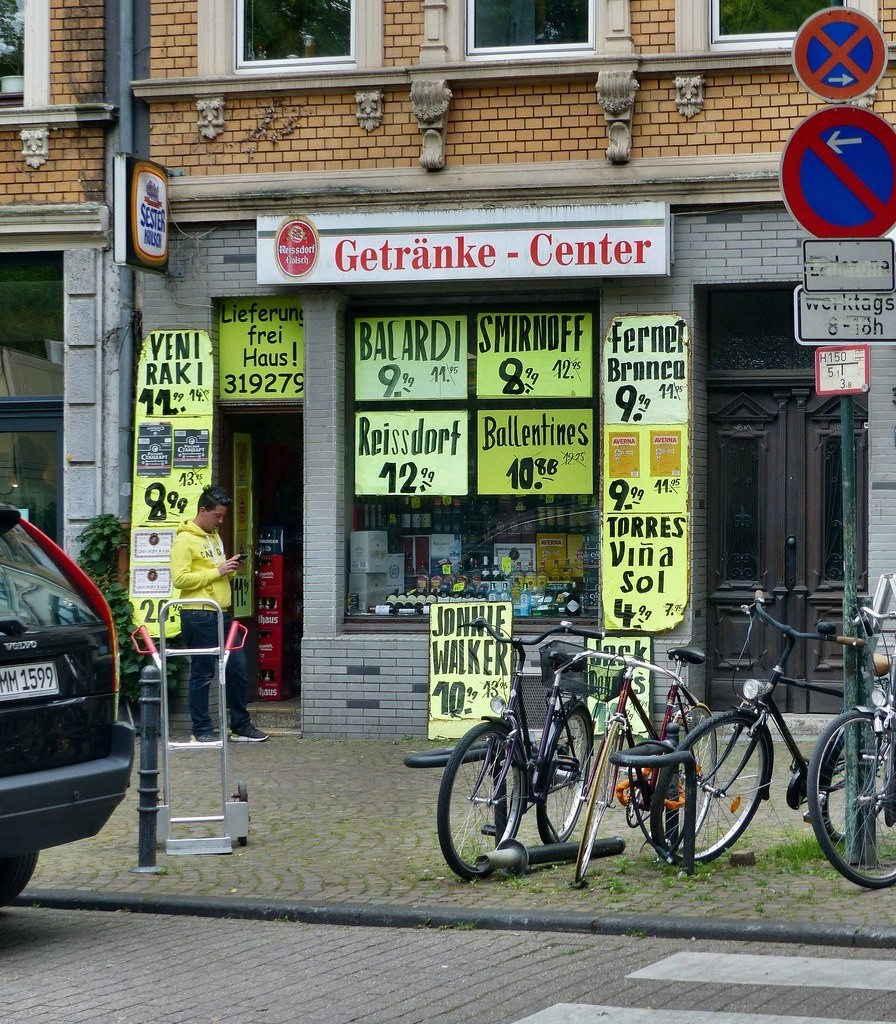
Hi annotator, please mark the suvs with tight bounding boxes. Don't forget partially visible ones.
[0,495,137,910]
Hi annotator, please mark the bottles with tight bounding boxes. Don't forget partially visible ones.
[401,496,432,534]
[500,583,511,601]
[491,556,502,581]
[257,597,277,609]
[368,588,437,615]
[562,559,573,581]
[536,561,549,591]
[512,578,522,604]
[520,583,532,617]
[565,582,583,616]
[535,494,590,533]
[257,669,275,682]
[551,560,561,581]
[481,556,492,581]
[524,560,537,591]
[487,583,501,601]
[513,562,525,591]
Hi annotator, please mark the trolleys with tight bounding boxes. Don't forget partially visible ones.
[131,597,252,858]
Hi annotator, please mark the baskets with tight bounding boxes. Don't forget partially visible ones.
[538,639,628,702]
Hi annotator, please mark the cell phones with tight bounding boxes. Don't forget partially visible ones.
[235,554,248,563]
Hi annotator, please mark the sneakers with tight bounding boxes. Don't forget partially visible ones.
[189,732,221,743]
[230,726,270,742]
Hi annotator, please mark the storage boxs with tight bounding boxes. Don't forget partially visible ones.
[349,530,405,613]
[253,525,302,700]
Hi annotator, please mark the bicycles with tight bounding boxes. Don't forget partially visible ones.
[806,605,896,889]
[649,590,889,870]
[436,616,606,883]
[552,646,718,889]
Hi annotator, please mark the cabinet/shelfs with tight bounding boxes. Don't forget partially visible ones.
[390,536,431,593]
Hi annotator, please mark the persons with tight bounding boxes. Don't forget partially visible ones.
[168,485,270,746]
